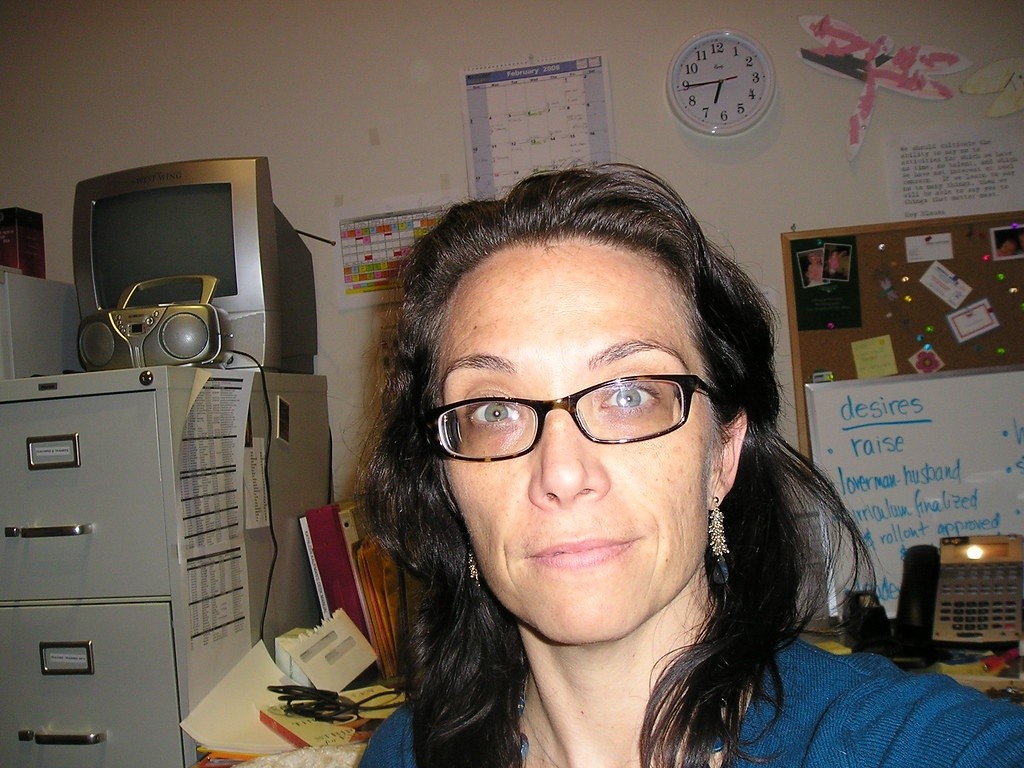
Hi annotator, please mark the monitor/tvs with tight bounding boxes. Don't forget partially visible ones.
[73,157,318,375]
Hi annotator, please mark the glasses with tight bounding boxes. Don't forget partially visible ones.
[415,374,723,462]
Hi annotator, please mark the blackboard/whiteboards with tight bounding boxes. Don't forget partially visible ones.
[805,367,1024,640]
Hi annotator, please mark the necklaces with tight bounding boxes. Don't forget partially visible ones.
[512,685,726,768]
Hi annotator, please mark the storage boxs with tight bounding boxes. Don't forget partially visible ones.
[275,627,316,690]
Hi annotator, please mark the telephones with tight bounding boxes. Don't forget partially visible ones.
[891,534,1023,655]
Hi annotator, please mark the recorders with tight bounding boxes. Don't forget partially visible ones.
[77,302,235,372]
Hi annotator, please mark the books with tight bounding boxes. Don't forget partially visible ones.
[197,497,422,768]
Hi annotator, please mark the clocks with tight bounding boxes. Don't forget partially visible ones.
[666,26,778,138]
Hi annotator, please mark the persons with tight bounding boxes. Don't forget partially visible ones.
[346,164,1024,768]
